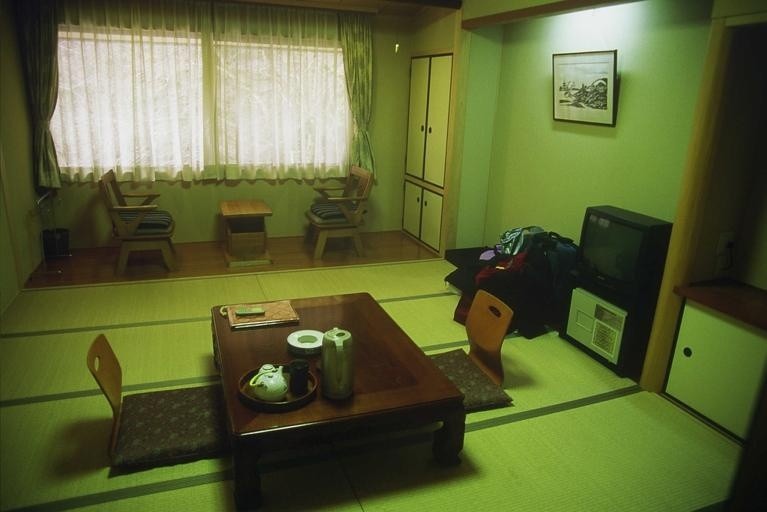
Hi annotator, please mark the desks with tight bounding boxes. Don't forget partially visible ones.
[211,292,466,512]
[218,199,273,268]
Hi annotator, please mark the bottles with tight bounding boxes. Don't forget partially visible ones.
[320,327,352,400]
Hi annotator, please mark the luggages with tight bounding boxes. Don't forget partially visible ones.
[537,231,579,305]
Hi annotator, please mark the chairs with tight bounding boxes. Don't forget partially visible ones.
[98,169,177,272]
[426,289,513,414]
[304,166,375,261]
[87,335,232,476]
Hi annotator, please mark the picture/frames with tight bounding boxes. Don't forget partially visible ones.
[553,50,617,127]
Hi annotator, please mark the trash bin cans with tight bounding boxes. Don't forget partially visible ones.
[42,229,70,266]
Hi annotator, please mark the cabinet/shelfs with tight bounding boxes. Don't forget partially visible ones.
[662,279,766,445]
[405,53,453,190]
[402,179,443,253]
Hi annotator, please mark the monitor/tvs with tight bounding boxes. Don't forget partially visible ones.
[574,205,673,298]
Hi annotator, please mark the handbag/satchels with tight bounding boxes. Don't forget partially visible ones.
[492,225,540,255]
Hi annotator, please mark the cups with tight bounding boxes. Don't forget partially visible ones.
[290,359,309,396]
[258,364,276,373]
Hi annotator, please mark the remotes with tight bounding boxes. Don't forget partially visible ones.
[235,308,265,315]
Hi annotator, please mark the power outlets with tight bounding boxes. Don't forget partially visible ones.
[717,234,735,256]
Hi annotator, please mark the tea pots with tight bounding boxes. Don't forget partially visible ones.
[249,365,289,401]
